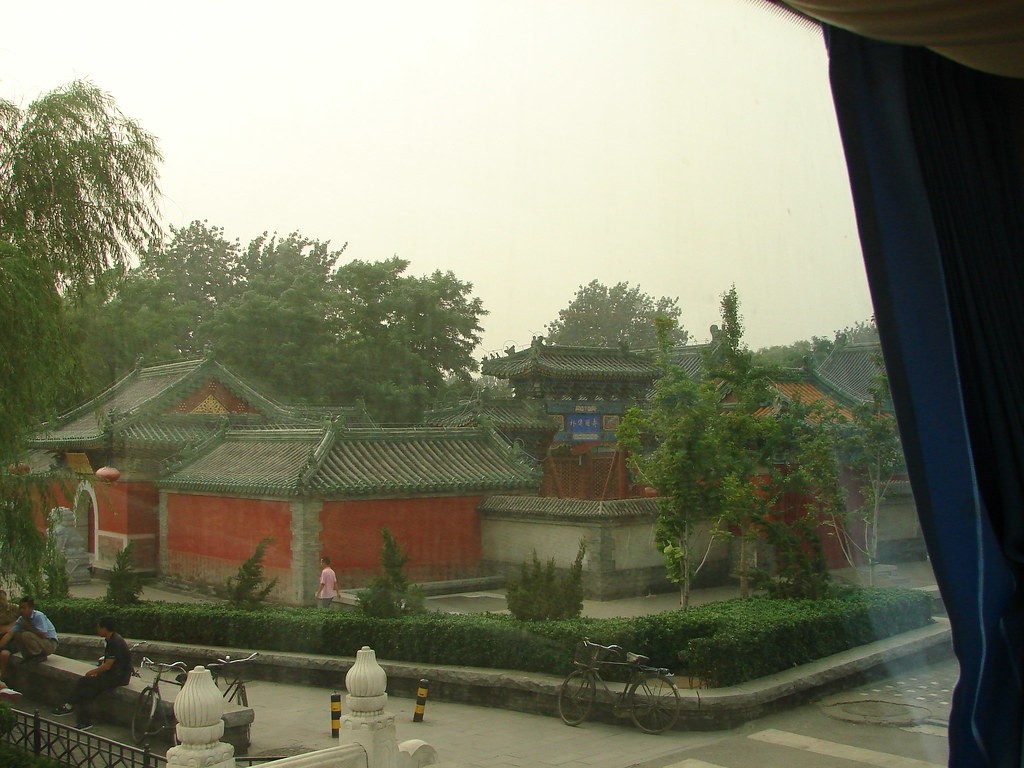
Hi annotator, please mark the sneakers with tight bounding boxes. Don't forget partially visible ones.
[0,688,23,700]
[74,720,93,731]
[50,705,74,716]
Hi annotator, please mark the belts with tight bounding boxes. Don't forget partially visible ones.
[46,638,58,645]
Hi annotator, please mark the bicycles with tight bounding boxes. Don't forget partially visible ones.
[206,651,261,743]
[558,636,682,736]
[131,656,188,747]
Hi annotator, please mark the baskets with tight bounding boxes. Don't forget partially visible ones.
[223,660,252,685]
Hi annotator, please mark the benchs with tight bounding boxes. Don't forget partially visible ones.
[10,649,254,759]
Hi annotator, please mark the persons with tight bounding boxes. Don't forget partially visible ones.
[314,555,341,608]
[0,596,59,664]
[0,590,23,700]
[50,616,142,731]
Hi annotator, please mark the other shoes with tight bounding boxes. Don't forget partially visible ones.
[24,657,44,666]
[17,658,32,664]
